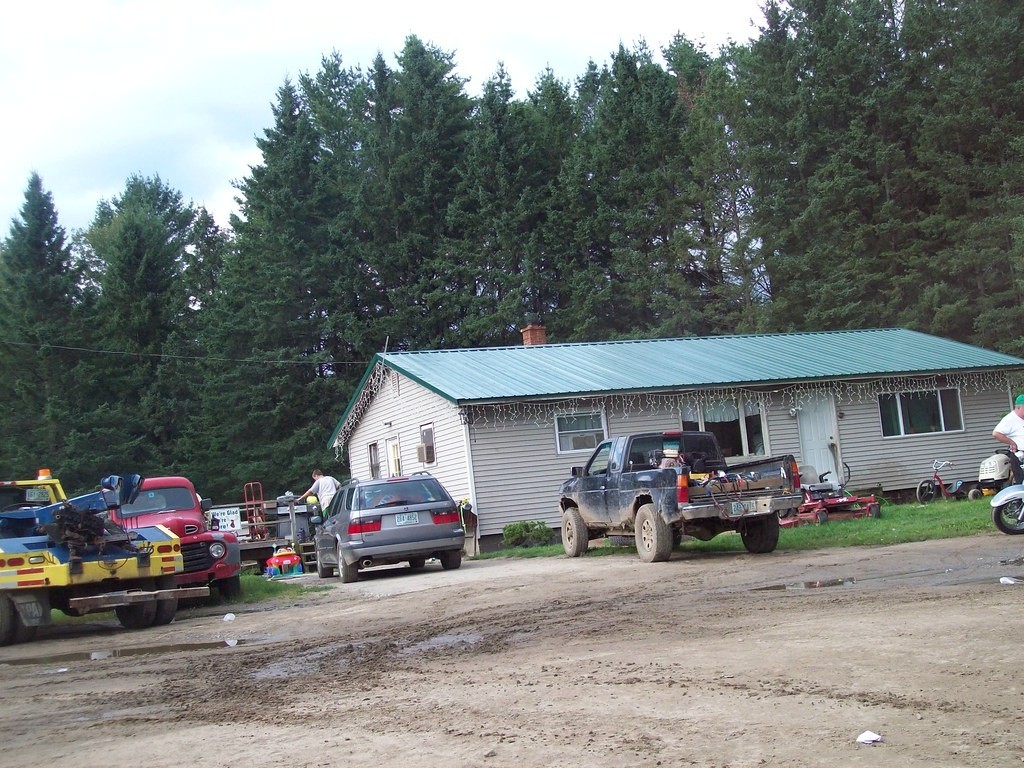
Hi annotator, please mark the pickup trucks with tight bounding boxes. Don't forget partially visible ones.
[557,430,804,563]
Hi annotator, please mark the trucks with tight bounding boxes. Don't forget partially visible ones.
[96,476,241,606]
[0,467,210,647]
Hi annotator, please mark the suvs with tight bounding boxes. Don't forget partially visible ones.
[309,471,466,584]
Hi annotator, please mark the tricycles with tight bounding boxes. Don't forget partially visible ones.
[916,458,983,505]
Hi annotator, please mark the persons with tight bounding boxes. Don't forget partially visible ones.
[992,395,1024,467]
[294,469,342,517]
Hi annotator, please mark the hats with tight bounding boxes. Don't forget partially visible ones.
[1015,394,1024,405]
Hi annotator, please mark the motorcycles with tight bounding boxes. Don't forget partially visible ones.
[990,483,1024,535]
